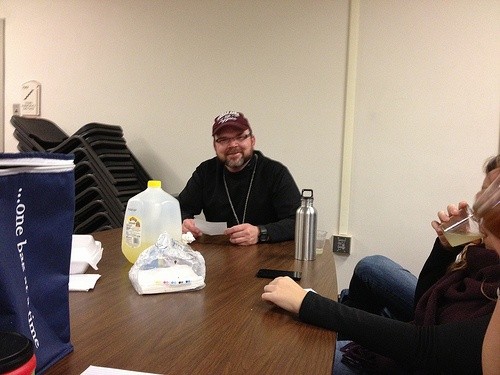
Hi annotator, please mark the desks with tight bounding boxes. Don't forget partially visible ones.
[37,227,338,375]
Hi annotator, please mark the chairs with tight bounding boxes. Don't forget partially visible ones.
[10,115,152,233]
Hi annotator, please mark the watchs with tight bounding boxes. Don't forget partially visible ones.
[259,227,268,243]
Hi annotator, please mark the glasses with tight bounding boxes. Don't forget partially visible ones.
[214,133,251,145]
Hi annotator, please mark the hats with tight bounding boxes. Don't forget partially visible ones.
[212,111,249,137]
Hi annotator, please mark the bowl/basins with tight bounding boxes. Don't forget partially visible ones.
[69,233,103,275]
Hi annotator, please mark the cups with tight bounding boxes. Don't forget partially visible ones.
[316,230,328,255]
[472,175,500,240]
[438,204,486,247]
[0,330,37,375]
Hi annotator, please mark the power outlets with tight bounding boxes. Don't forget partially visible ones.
[332,234,352,255]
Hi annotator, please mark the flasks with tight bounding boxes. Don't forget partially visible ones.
[294,188,317,261]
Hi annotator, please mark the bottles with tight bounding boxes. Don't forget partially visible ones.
[121,179,183,263]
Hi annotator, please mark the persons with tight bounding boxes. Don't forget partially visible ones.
[177,110,301,245]
[263,155,500,375]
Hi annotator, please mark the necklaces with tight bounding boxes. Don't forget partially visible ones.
[223,154,259,226]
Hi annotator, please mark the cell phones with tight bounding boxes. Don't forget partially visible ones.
[256,269,295,278]
